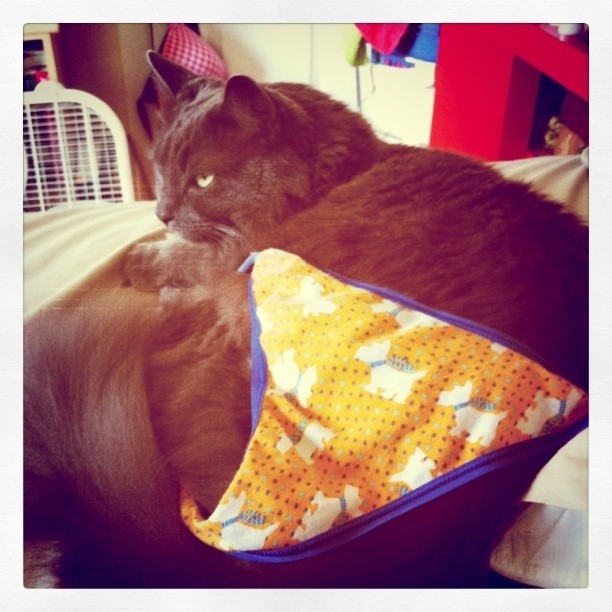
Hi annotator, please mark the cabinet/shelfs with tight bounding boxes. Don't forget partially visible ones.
[428,27,588,160]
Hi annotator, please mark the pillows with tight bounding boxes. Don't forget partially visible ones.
[180,244,587,561]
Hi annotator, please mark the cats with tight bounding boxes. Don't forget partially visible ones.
[119,48,589,543]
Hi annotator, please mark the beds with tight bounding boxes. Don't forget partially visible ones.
[25,89,587,589]
